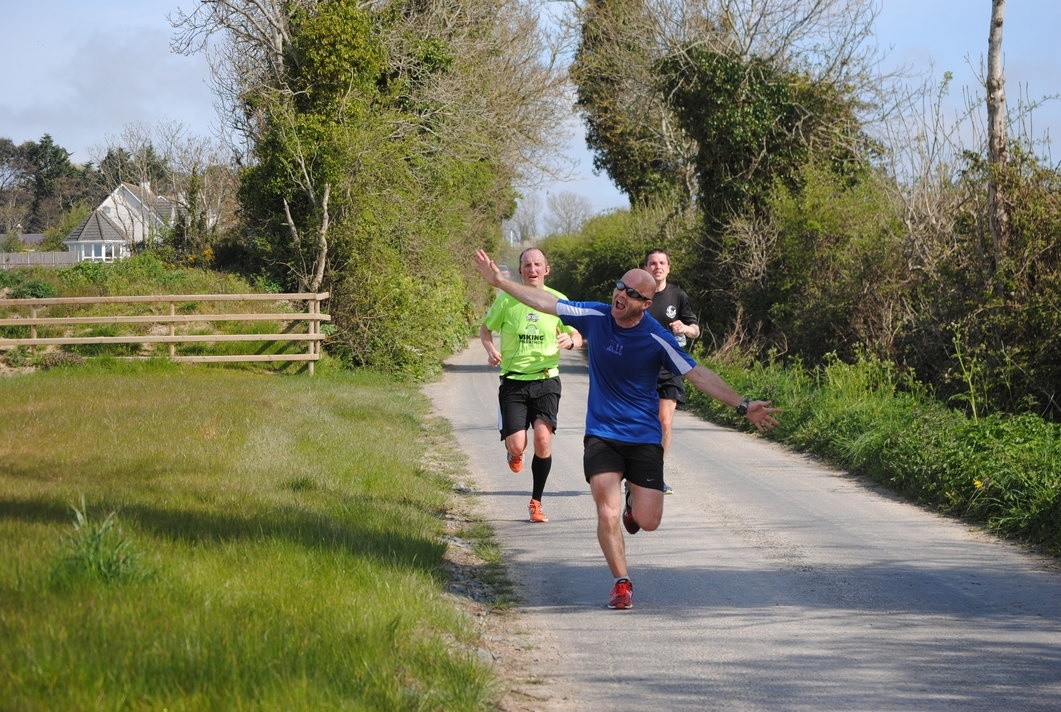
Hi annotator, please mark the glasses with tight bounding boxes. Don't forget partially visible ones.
[616,280,653,302]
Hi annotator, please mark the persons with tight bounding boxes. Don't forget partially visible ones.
[473,246,782,610]
[634,250,700,495]
[479,247,583,523]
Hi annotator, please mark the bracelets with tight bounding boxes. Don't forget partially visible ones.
[568,340,574,350]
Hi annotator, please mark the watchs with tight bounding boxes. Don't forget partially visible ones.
[736,397,750,416]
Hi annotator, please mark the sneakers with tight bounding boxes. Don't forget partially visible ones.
[527,498,548,522]
[607,578,634,608]
[623,486,640,535]
[507,451,525,473]
